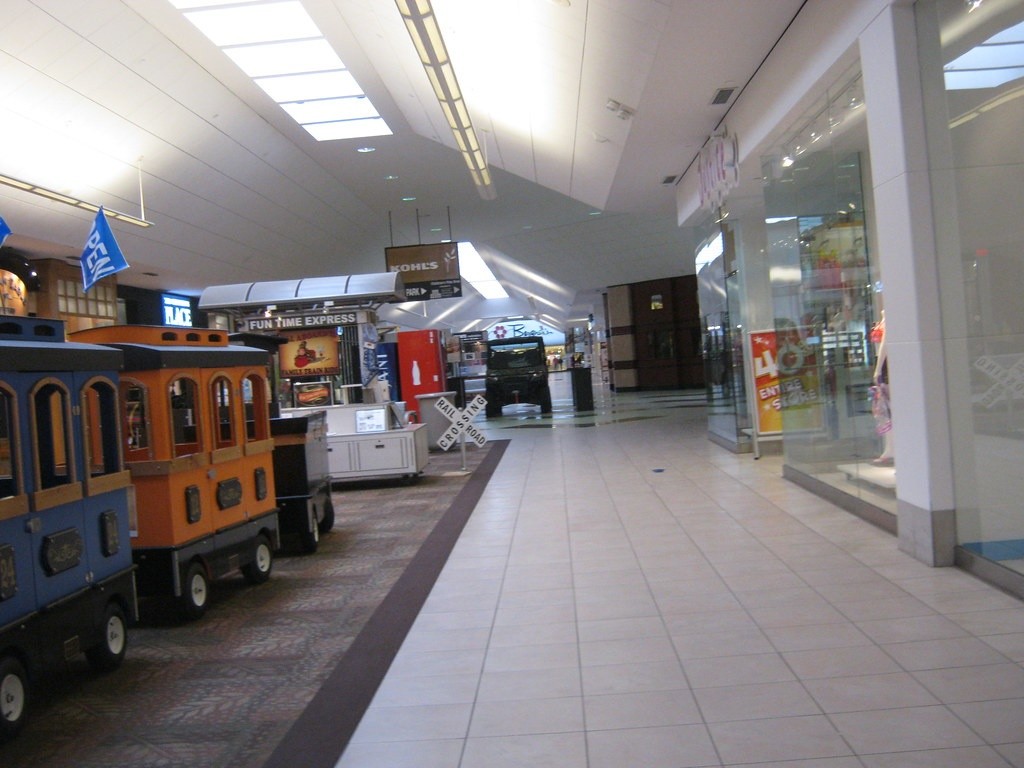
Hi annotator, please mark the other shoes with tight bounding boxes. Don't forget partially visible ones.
[873,455,894,463]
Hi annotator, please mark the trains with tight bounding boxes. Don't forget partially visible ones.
[0,315,335,741]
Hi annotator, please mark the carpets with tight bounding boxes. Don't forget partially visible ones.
[0,438,512,768]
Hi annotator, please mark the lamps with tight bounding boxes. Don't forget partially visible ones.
[322,301,334,314]
[265,305,277,317]
[0,155,155,229]
[393,0,497,202]
[442,240,509,300]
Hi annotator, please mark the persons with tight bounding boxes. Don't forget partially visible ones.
[553,356,563,370]
[870,310,893,463]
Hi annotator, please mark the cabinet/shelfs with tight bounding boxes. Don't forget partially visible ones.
[459,357,487,402]
[279,404,431,484]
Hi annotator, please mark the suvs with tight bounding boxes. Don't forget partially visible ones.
[486,334,551,418]
